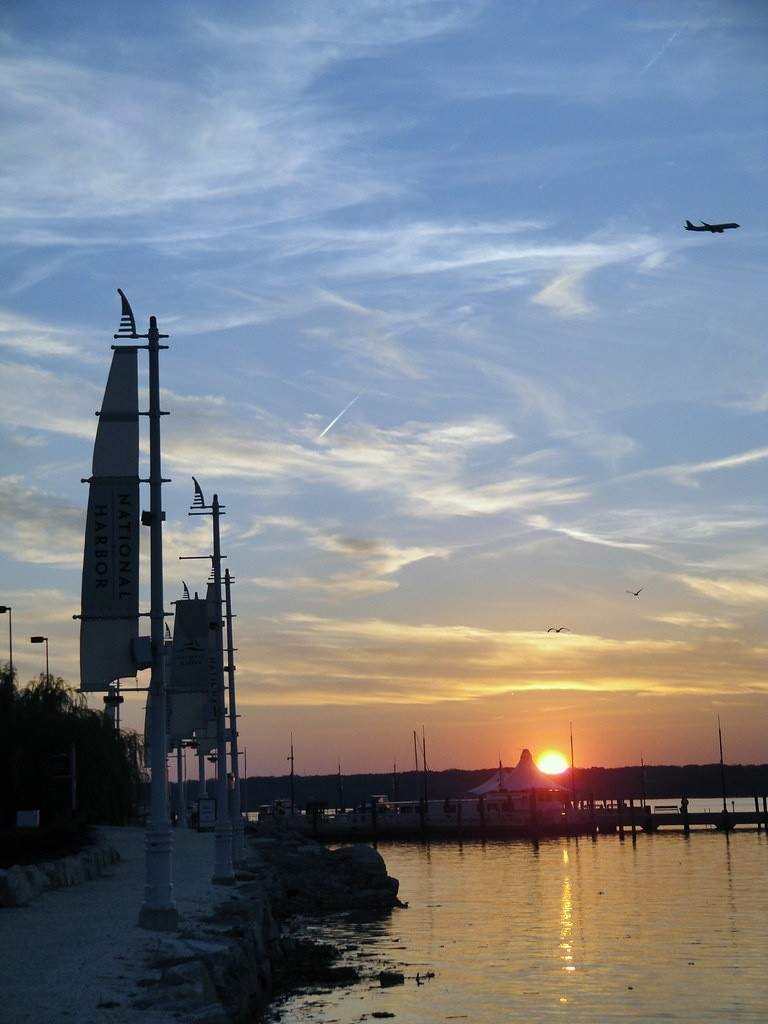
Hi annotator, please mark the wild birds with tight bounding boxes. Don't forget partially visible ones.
[625,588,644,596]
[547,627,569,633]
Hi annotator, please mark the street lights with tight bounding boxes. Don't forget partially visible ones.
[31,637,48,690]
[0,606,12,673]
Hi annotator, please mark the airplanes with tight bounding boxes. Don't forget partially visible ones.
[683,219,740,233]
